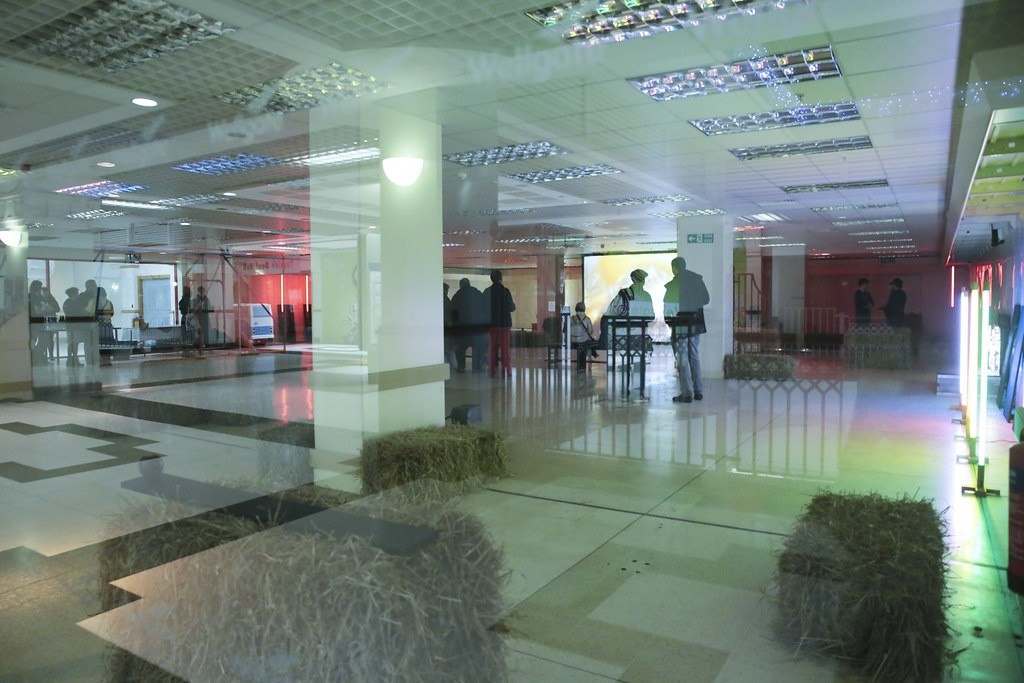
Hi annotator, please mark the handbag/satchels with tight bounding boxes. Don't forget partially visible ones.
[185,307,202,330]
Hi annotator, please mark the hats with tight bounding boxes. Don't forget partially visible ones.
[890,278,902,287]
[631,269,648,278]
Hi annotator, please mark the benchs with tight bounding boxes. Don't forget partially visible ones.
[548,342,606,369]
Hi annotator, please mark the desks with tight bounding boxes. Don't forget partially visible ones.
[121,325,182,357]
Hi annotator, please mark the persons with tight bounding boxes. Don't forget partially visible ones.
[878,279,907,326]
[854,278,874,327]
[608,269,656,403]
[482,271,516,378]
[179,285,214,358]
[28,279,60,366]
[443,283,451,364]
[451,278,483,372]
[63,279,114,368]
[663,257,710,403]
[570,302,599,369]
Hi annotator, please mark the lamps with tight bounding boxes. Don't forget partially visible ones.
[450,402,484,426]
[0,231,22,247]
[381,155,423,186]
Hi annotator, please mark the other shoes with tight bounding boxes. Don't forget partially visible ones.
[73,359,84,366]
[579,366,585,371]
[694,394,703,399]
[619,394,628,401]
[594,354,599,358]
[66,360,75,366]
[673,396,692,403]
[632,396,650,402]
